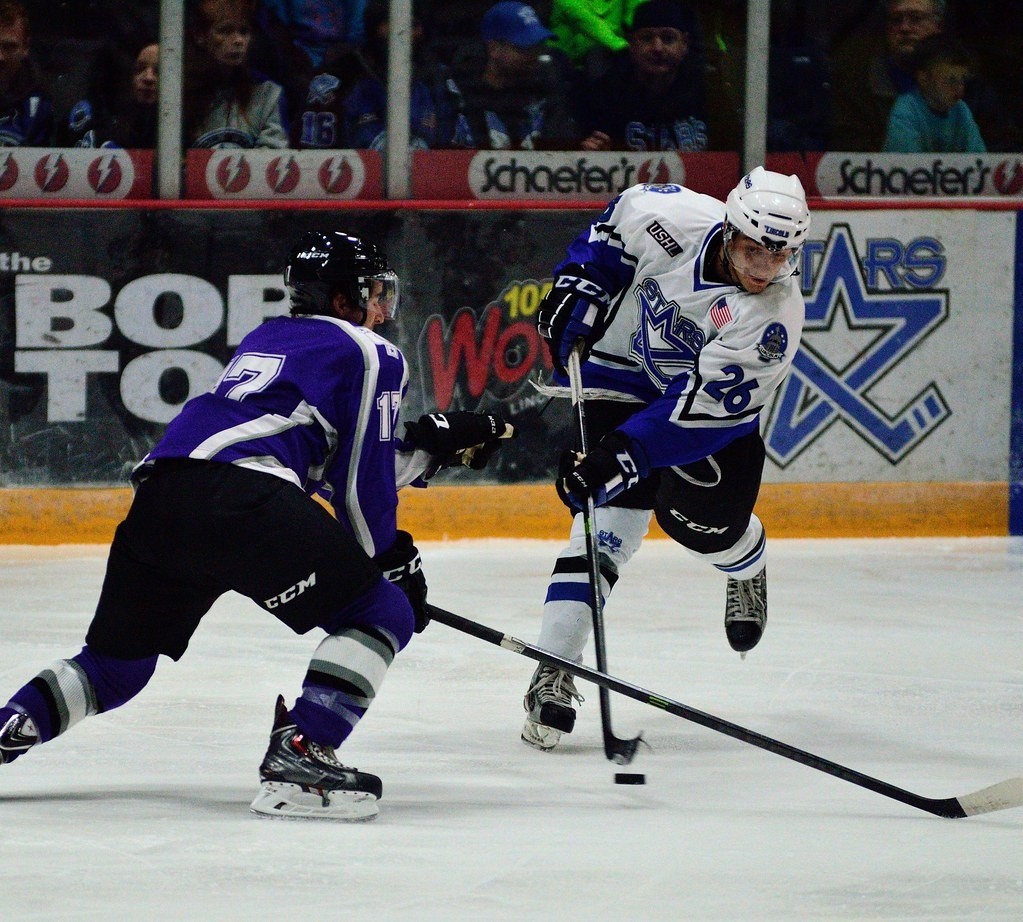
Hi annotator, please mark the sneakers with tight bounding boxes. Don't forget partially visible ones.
[519,655,584,751]
[725,567,769,664]
[249,695,385,823]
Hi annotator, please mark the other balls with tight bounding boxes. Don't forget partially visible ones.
[613,773,645,786]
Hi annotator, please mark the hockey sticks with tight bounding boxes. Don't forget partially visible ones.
[418,603,1020,820]
[564,343,644,766]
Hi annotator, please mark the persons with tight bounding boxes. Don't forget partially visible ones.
[0,0,1023,151]
[0,229,431,824]
[519,166,813,752]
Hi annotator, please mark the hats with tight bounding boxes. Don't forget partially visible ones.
[911,33,968,71]
[633,0,690,33]
[481,1,559,49]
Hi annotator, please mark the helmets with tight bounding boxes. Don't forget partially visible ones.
[723,166,811,283]
[284,231,399,321]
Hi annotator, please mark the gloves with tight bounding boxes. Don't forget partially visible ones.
[537,263,615,376]
[556,428,649,512]
[404,411,507,488]
[373,531,430,634]
